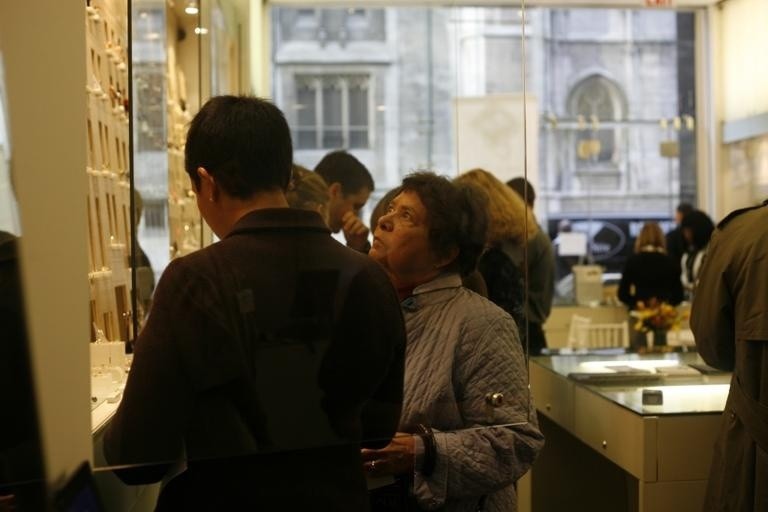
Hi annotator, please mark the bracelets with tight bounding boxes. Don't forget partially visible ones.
[414,422,435,479]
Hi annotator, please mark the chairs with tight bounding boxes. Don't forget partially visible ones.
[566,315,630,349]
[572,264,618,306]
[646,329,696,346]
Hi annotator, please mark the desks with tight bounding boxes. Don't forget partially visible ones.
[515,346,734,512]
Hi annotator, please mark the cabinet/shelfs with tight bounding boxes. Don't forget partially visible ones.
[0,0,252,512]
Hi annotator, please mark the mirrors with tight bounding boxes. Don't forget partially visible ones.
[131,0,172,338]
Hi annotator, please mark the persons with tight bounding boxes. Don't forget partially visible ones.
[618,222,683,351]
[132,188,155,307]
[549,219,589,280]
[689,199,768,512]
[105,93,405,512]
[457,169,542,311]
[681,211,714,279]
[505,178,554,356]
[287,164,330,229]
[666,202,694,275]
[358,168,545,511]
[313,150,373,254]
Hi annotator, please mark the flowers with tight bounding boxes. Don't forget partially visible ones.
[629,297,691,333]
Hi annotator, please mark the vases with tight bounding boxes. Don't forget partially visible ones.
[653,331,665,345]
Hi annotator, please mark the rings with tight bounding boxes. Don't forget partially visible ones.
[369,460,377,473]
